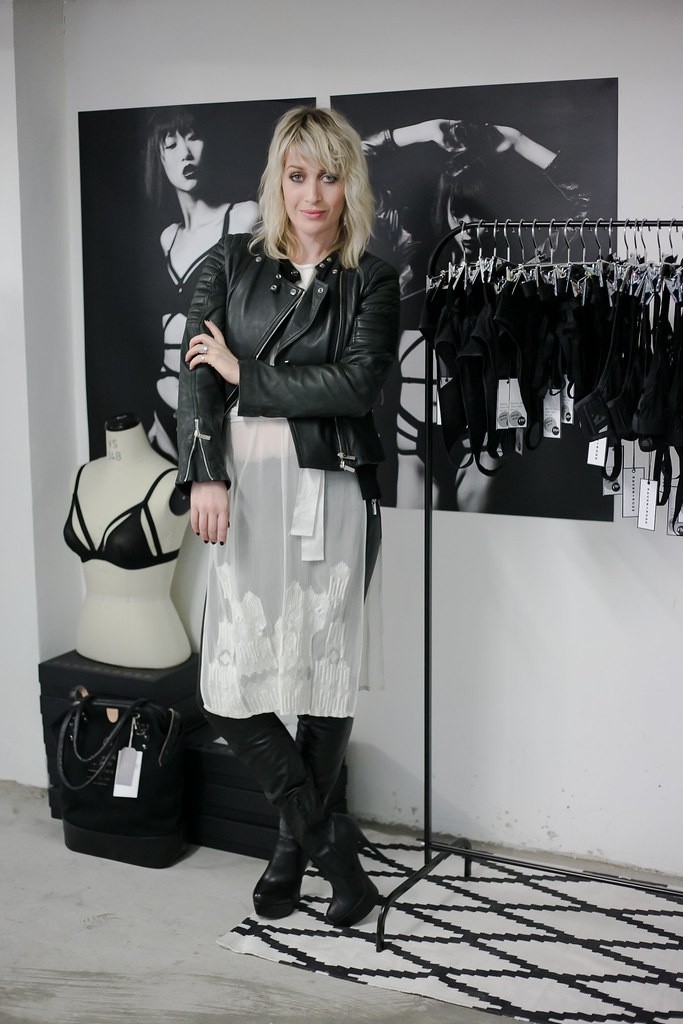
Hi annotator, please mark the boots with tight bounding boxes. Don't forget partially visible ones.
[203,709,378,928]
[253,715,354,918]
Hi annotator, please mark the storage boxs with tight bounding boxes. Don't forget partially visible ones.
[36,654,348,859]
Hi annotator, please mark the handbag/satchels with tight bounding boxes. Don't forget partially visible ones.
[57,700,198,868]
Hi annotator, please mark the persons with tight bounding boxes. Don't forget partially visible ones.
[169,106,403,926]
[360,119,611,329]
[140,106,262,464]
[64,416,193,669]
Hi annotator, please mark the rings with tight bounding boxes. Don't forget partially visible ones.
[199,344,208,353]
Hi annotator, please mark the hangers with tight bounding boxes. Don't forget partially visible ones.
[426,219,683,305]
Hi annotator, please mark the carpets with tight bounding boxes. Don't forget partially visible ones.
[216,828,683,1024]
